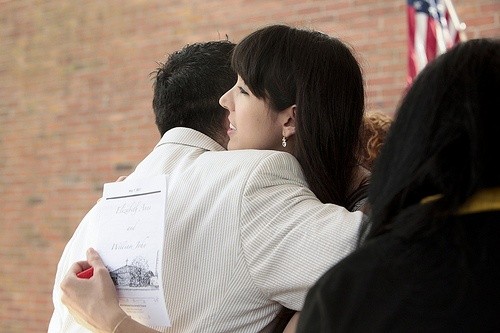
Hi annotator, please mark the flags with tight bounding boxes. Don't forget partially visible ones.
[405,0,466,86]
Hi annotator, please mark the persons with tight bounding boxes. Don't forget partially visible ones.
[296,38,500,333]
[48,34,375,333]
[60,25,372,333]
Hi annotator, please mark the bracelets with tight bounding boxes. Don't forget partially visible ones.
[112,314,132,333]
